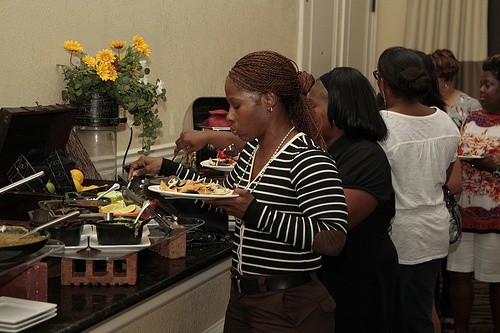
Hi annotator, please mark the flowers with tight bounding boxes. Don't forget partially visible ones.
[57,35,167,158]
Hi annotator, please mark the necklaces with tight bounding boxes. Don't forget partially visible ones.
[235,125,295,192]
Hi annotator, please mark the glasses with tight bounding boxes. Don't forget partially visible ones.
[372,69,381,80]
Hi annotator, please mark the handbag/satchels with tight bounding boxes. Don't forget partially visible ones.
[442,185,464,245]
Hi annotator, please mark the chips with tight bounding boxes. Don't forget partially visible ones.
[159,177,213,193]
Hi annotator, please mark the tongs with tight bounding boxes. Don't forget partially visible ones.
[139,178,182,221]
[28,208,113,223]
[121,185,174,236]
[37,192,111,210]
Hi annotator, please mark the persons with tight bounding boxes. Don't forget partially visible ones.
[127,53,349,333]
[373,47,500,333]
[174,66,403,333]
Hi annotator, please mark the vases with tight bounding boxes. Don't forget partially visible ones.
[76,93,119,118]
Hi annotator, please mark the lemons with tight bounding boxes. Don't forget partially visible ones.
[69,169,100,192]
[45,183,55,193]
[100,203,142,213]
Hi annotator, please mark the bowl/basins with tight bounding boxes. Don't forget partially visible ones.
[28,217,87,247]
[93,216,156,245]
[99,204,141,216]
[0,223,51,265]
[97,191,122,199]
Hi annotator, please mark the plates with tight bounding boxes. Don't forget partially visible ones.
[39,218,158,260]
[458,155,485,160]
[0,296,57,333]
[147,185,240,198]
[200,158,234,171]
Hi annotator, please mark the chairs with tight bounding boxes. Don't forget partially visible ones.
[193,97,230,170]
